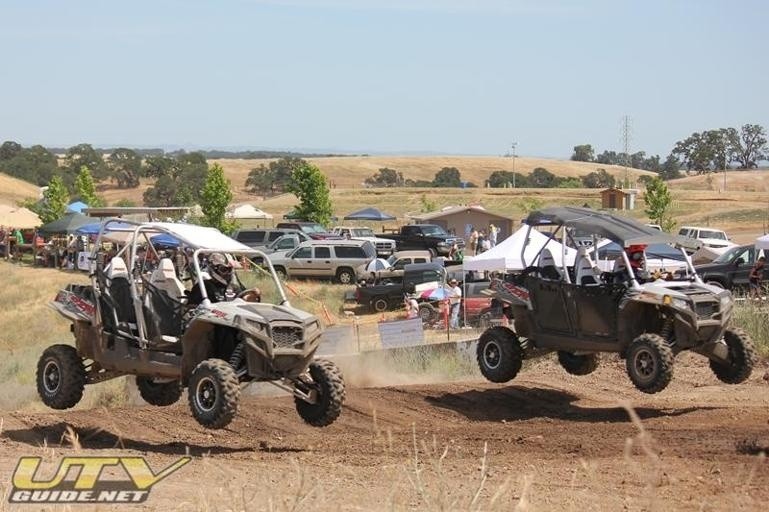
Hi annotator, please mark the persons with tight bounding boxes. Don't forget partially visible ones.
[748,257,766,305]
[615,244,665,285]
[182,254,261,333]
[368,272,377,284]
[448,278,462,332]
[467,224,497,255]
[9,227,24,261]
[0,225,10,260]
[32,233,167,271]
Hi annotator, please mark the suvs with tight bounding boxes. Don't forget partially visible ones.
[36,220,345,428]
[477,207,753,394]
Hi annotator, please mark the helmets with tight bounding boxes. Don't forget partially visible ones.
[207,252,233,286]
[624,243,648,268]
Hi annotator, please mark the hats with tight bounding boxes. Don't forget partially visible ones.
[449,279,458,286]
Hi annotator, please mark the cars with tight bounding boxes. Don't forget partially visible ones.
[694,245,769,294]
[233,223,492,321]
[567,229,594,248]
[677,225,732,255]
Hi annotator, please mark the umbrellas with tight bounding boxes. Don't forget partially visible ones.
[364,256,392,282]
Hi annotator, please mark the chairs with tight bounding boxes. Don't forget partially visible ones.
[536,245,625,288]
[103,256,210,340]
